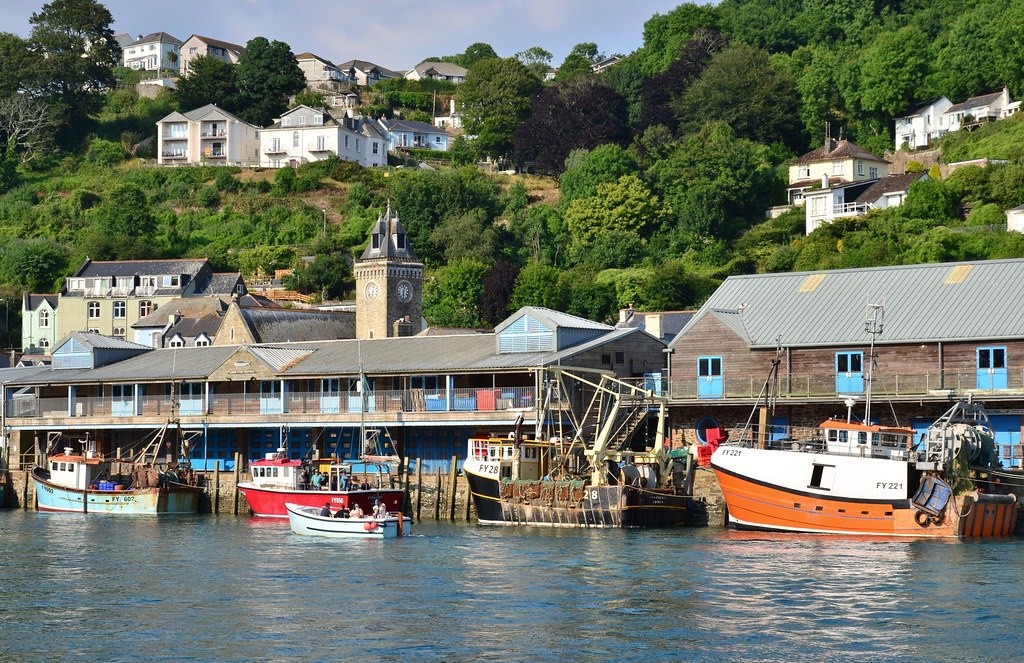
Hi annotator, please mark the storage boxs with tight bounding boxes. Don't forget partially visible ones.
[93,480,119,490]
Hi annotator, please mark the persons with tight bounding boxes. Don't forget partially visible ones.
[373,498,386,517]
[320,502,332,517]
[349,503,364,518]
[297,469,370,491]
[334,505,350,518]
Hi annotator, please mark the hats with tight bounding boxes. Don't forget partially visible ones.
[301,469,306,472]
[315,469,319,472]
[354,504,358,507]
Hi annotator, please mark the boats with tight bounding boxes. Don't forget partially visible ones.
[463,362,692,527]
[235,359,406,520]
[30,371,205,516]
[708,296,1024,540]
[285,501,412,539]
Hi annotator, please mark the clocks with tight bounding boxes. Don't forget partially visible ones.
[367,284,378,299]
[398,284,409,298]
